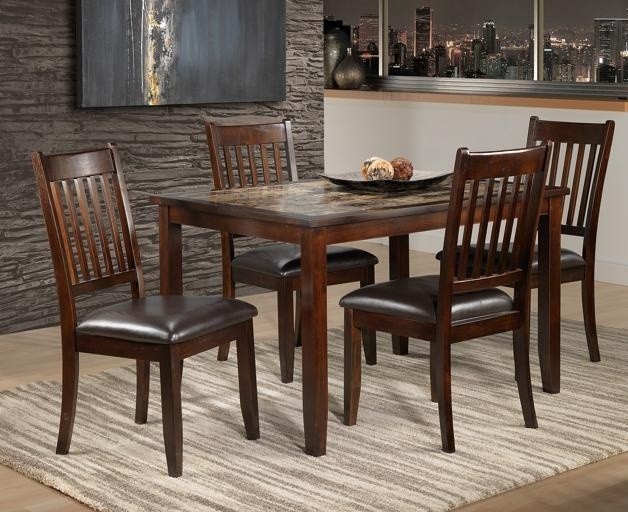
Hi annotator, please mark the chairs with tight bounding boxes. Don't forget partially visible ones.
[340,137,553,454]
[205,117,379,384]
[31,139,260,477]
[433,115,616,363]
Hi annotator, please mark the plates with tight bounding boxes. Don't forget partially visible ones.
[314,169,454,191]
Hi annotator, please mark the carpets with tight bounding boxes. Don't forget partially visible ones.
[0,313,628,512]
